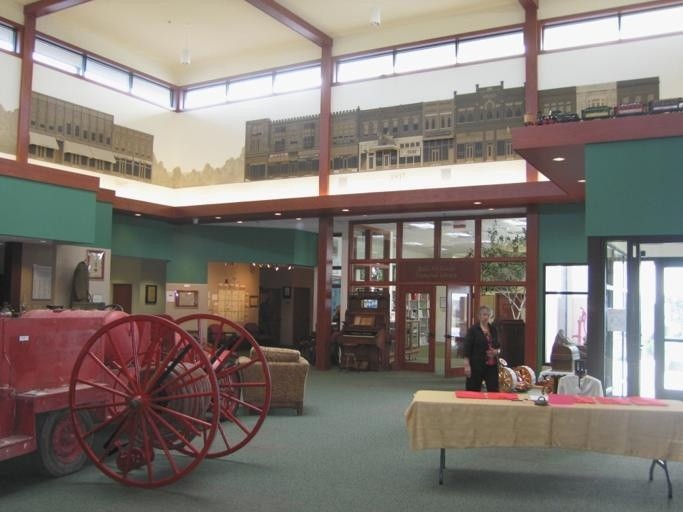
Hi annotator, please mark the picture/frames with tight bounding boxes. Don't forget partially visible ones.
[86,250,103,280]
[146,286,156,304]
[282,287,291,298]
[249,296,258,307]
[175,289,198,310]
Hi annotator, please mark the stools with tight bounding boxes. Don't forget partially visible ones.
[341,352,358,377]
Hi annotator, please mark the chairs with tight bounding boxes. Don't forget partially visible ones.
[235,346,310,419]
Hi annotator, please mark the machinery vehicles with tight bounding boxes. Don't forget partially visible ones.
[0,306,243,479]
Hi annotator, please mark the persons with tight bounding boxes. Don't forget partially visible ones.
[377,128,396,144]
[332,303,341,323]
[462,306,504,392]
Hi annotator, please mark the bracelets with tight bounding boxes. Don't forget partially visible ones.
[498,349,500,355]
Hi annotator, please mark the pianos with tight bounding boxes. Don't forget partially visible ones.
[336,290,392,371]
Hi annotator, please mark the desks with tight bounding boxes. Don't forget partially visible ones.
[411,388,683,499]
[539,367,576,395]
